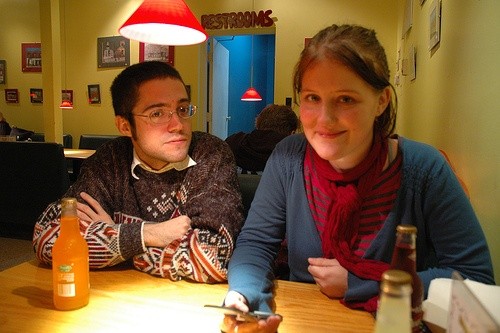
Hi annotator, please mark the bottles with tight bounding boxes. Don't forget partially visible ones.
[373,269,413,333]
[52,197,90,310]
[387,225,424,333]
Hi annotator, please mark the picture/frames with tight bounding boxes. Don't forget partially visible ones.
[4,88,19,103]
[62,90,74,106]
[30,88,44,103]
[394,0,442,87]
[0,59,6,84]
[139,43,175,66]
[88,84,102,104]
[21,42,42,73]
[96,36,132,68]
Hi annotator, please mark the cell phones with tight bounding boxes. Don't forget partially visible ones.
[205,304,259,323]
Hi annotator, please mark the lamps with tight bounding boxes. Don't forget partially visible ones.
[118,0,209,47]
[240,0,262,102]
[60,0,74,110]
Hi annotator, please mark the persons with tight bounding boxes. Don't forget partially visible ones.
[33,61,244,285]
[218,24,496,333]
[224,104,300,176]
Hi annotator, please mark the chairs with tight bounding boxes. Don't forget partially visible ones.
[78,134,120,149]
[0,140,81,239]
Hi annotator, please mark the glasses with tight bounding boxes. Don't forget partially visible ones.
[130,104,199,124]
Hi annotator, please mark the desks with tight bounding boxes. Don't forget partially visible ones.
[63,149,96,160]
[0,258,376,333]
